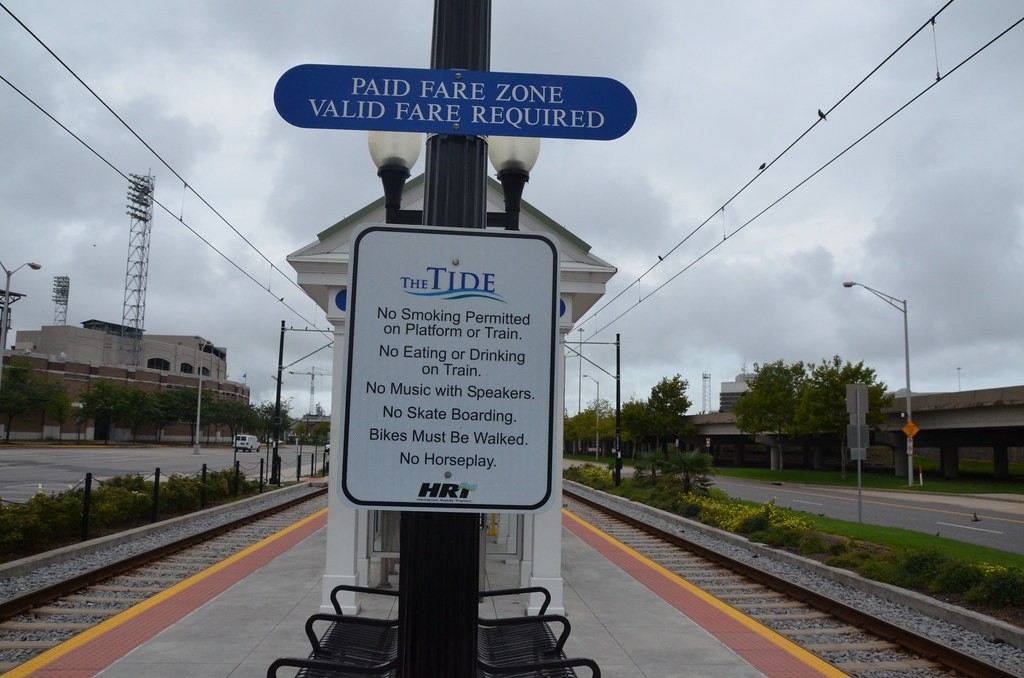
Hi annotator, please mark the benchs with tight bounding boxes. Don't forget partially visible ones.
[266,584,399,678]
[477,586,601,678]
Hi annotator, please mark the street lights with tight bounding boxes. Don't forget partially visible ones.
[0,259,41,382]
[843,280,914,489]
[360,117,545,678]
[577,326,585,453]
[582,374,600,462]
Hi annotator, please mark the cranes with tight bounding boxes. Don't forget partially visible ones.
[288,366,334,416]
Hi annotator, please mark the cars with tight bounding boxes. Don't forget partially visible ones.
[265,438,284,444]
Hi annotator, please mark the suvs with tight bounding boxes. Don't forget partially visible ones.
[232,435,261,453]
